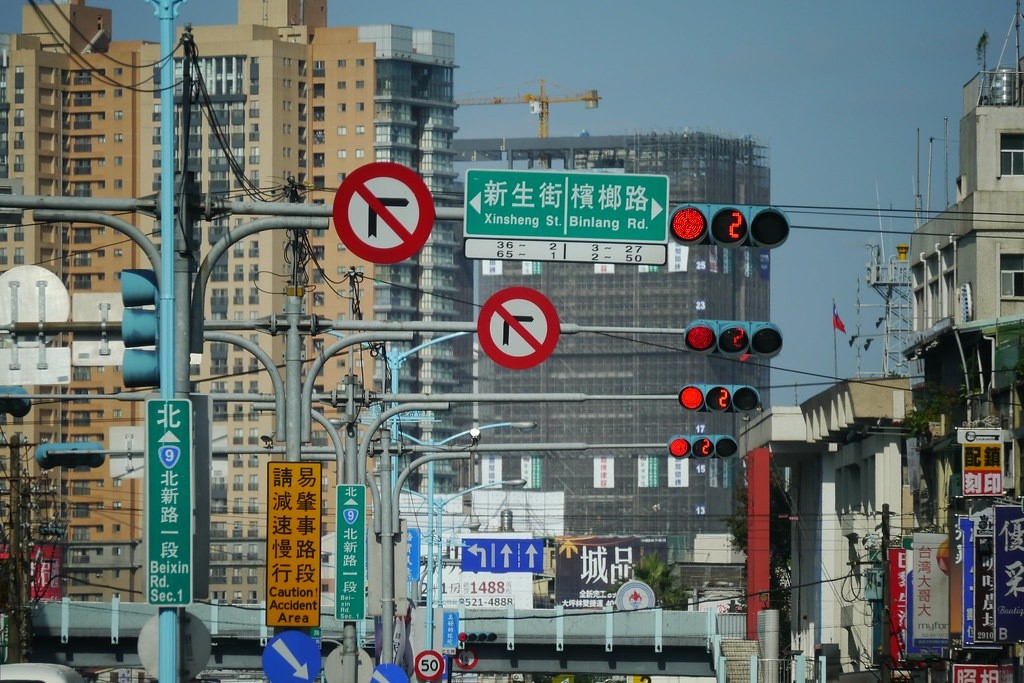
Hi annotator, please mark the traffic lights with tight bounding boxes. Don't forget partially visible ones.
[34,440,104,470]
[668,436,746,464]
[2,385,31,421]
[677,386,760,415]
[119,263,169,390]
[668,204,791,248]
[683,322,781,359]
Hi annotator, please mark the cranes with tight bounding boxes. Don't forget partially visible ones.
[453,79,604,138]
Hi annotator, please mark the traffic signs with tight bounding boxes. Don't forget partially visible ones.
[465,168,671,266]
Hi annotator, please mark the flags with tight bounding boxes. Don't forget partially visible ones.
[833,301,847,335]
